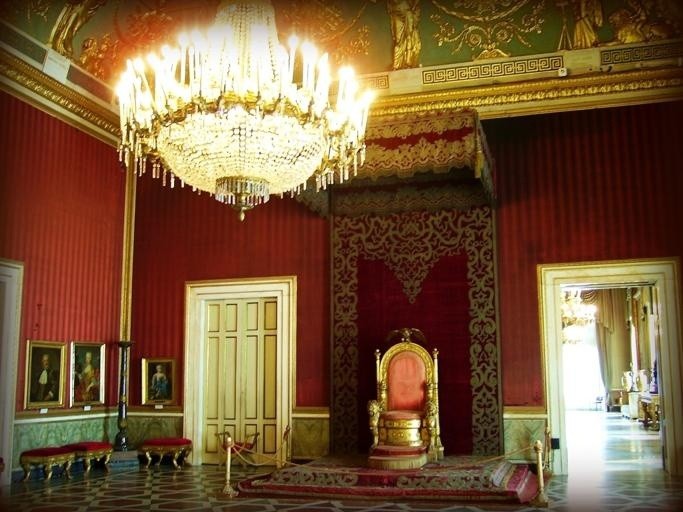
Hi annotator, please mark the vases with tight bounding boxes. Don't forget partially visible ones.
[620,368,648,393]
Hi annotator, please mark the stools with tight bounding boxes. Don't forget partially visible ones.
[19,437,193,482]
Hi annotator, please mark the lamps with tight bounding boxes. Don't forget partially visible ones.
[114,2,379,221]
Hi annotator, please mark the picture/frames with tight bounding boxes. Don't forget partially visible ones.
[22,338,175,411]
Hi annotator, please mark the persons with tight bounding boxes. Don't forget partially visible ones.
[31,351,57,401]
[151,364,169,400]
[626,0,649,43]
[75,351,98,401]
[568,0,601,50]
[383,0,426,71]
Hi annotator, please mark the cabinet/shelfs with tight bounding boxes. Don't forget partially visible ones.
[291,405,330,460]
[502,404,548,464]
[607,388,628,412]
[641,394,661,431]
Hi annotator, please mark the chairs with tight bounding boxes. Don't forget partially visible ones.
[367,328,443,469]
[215,430,260,468]
